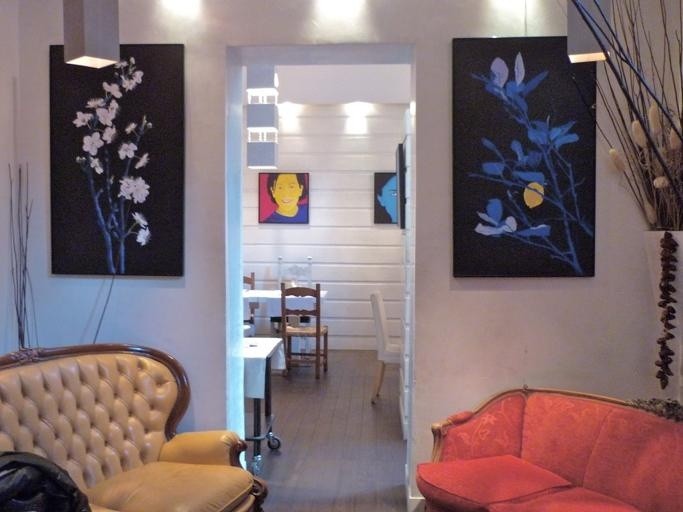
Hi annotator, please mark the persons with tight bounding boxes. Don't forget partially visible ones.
[261,173,308,224]
[376,174,398,224]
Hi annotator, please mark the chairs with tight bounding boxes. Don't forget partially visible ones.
[369,290,401,404]
[242,272,255,324]
[280,282,328,379]
[269,257,313,360]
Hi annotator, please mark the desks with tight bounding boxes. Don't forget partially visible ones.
[243,336,280,477]
[243,287,329,368]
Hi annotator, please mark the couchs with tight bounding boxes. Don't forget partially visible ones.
[415,386,683,512]
[0,342,268,511]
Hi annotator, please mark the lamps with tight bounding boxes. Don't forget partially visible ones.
[62,0,119,69]
[246,65,279,170]
[566,0,613,65]
